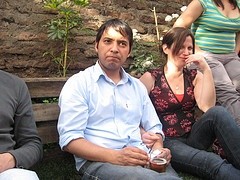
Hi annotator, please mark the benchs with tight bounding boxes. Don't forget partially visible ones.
[20,76,140,180]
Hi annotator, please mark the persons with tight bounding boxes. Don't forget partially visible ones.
[0,70,43,180]
[140,27,240,180]
[57,19,184,180]
[172,0,240,129]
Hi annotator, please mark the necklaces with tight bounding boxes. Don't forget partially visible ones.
[173,82,182,90]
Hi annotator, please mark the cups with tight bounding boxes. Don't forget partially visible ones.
[151,149,167,173]
